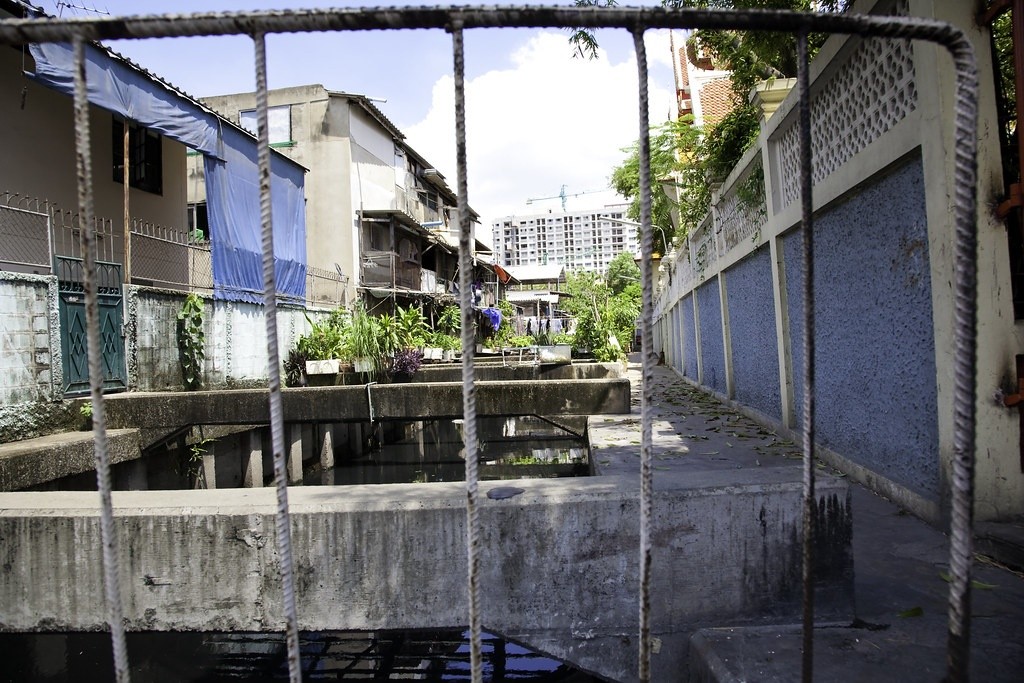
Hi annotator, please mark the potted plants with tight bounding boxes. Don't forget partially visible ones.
[423,331,463,362]
[474,336,572,361]
[353,302,378,385]
[298,323,343,375]
[577,335,591,353]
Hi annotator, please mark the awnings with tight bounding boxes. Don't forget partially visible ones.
[424,232,459,255]
[0,0,309,173]
[475,237,521,287]
[355,209,436,237]
